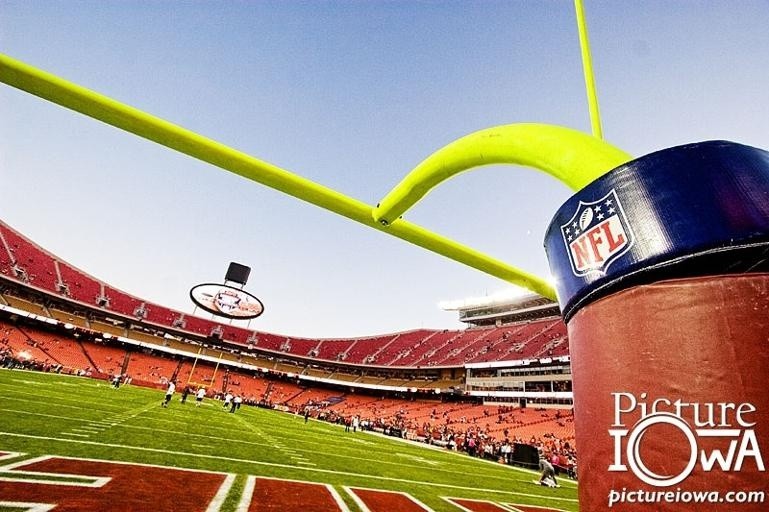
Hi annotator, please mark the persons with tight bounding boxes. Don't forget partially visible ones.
[0,244,577,479]
[536,460,560,488]
